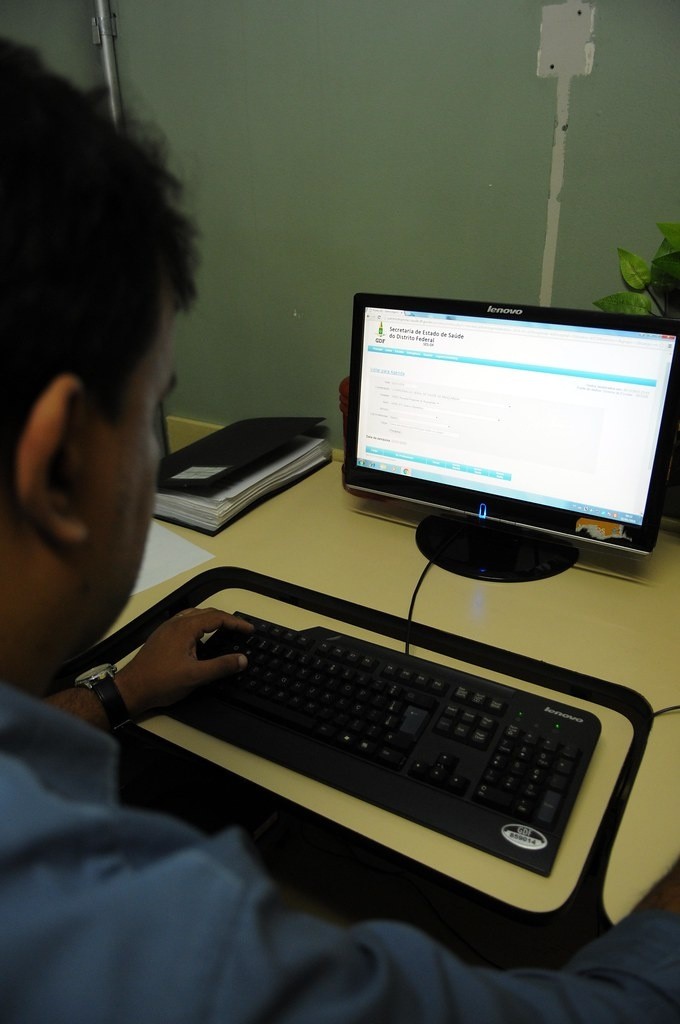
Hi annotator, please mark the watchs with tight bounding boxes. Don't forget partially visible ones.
[74,662,131,732]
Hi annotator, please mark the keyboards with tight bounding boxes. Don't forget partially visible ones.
[166,610,602,876]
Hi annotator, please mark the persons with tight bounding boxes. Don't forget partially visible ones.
[0,40,680,1024]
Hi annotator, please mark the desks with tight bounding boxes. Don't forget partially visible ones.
[98,419,680,963]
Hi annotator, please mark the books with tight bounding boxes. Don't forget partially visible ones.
[144,406,333,537]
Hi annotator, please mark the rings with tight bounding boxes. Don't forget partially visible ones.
[175,613,184,616]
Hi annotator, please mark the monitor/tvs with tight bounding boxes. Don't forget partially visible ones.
[344,293,680,583]
